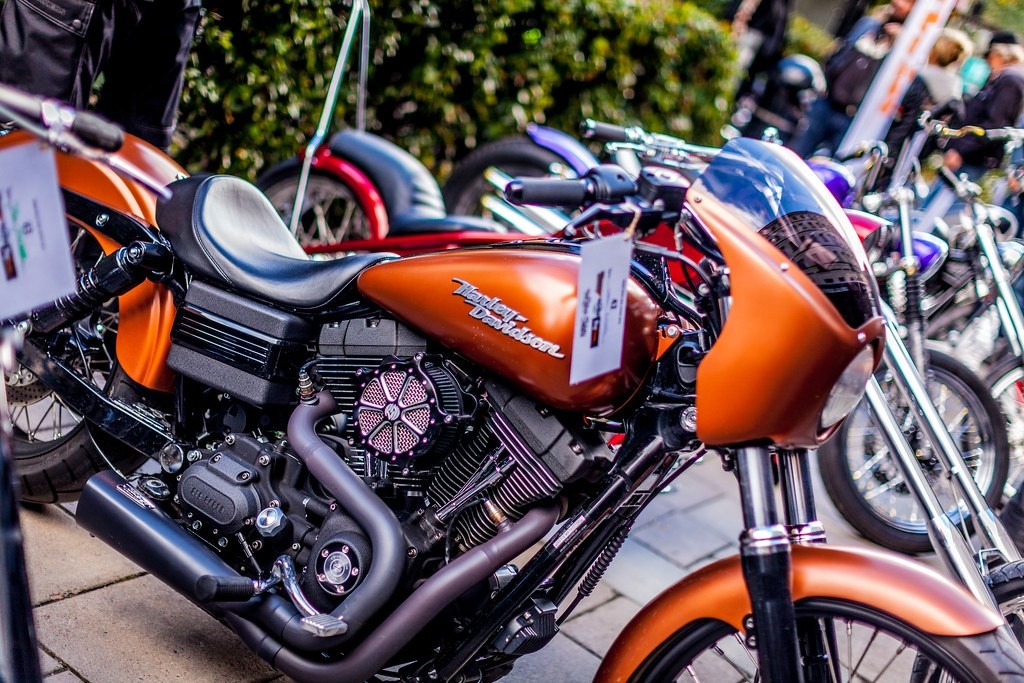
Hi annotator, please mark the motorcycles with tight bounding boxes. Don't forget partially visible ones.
[2,85,1024,683]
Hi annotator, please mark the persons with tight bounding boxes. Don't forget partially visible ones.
[715,0,1024,218]
[0,0,204,148]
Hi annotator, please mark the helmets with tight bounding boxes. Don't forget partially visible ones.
[775,53,827,111]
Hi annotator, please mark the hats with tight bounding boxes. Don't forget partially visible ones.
[983,32,1017,59]
